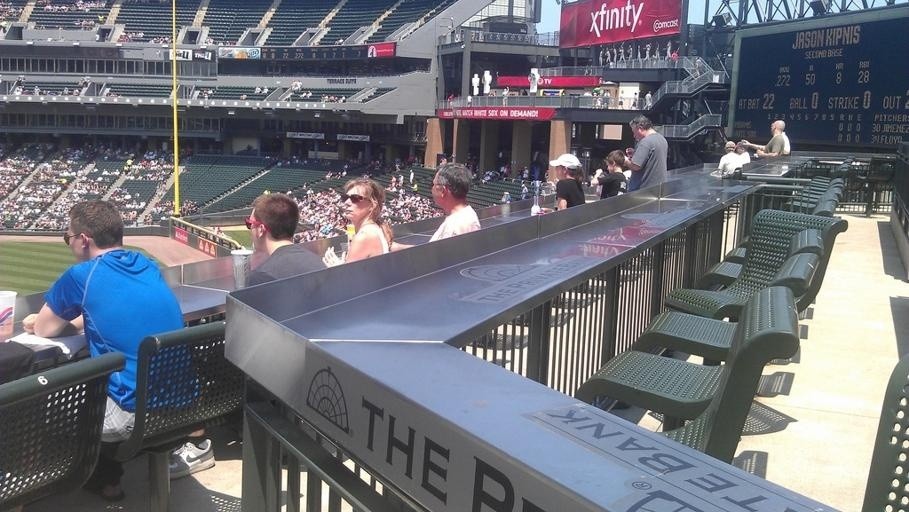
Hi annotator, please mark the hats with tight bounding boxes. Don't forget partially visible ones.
[549,153,583,170]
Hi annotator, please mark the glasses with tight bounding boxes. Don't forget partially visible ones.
[242,216,263,228]
[339,192,373,204]
[62,231,91,245]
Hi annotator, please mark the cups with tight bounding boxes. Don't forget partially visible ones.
[0,291,17,336]
[230,249,254,290]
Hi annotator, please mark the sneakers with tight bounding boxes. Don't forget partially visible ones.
[168,439,217,480]
[82,477,125,502]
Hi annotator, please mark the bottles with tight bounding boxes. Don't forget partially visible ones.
[501,192,511,218]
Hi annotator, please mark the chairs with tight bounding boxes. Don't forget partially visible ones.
[376,165,533,215]
[2,320,244,506]
[1,146,372,232]
[1,79,395,106]
[1,3,453,50]
[862,353,908,509]
[784,157,896,218]
[578,208,848,465]
